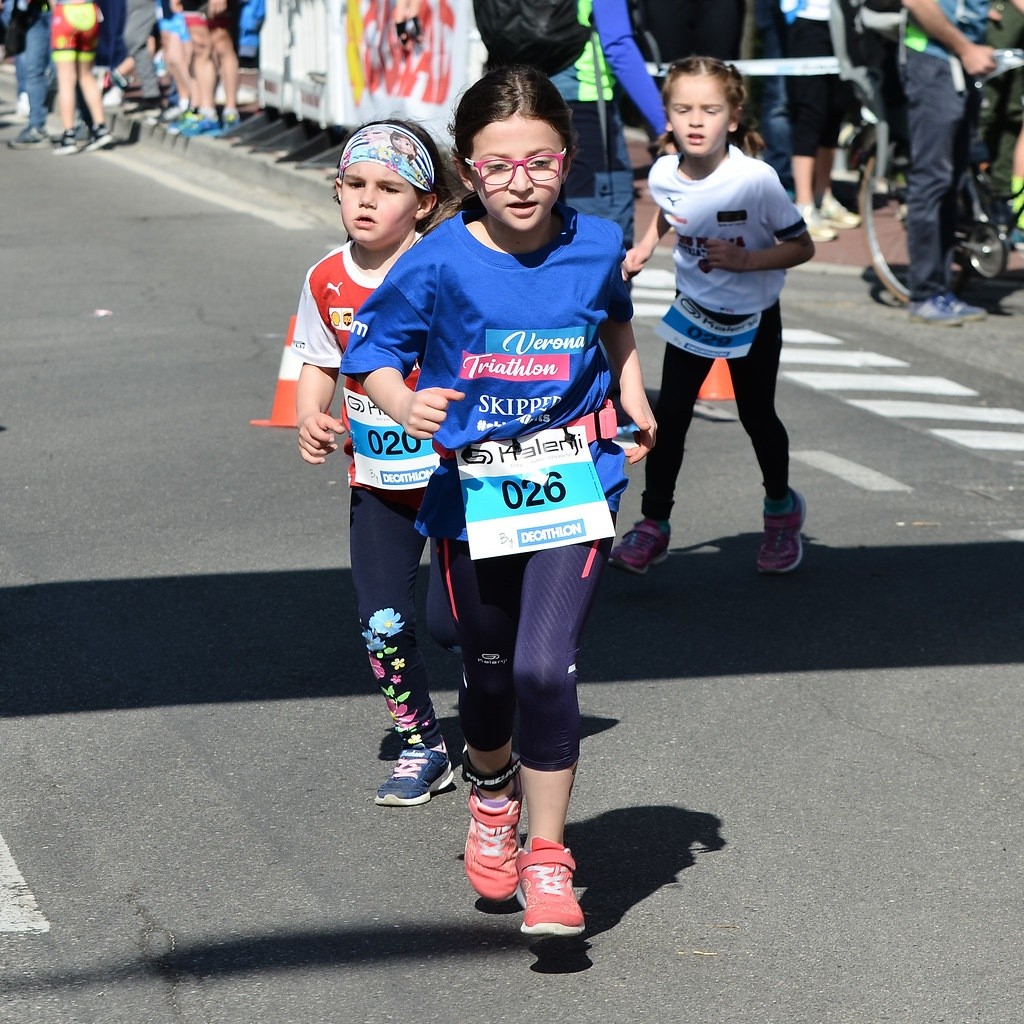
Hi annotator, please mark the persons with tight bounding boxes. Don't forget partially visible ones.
[290,121,454,806]
[607,56,816,573]
[551,1,667,290]
[0,0,239,155]
[642,0,1024,325]
[339,66,657,934]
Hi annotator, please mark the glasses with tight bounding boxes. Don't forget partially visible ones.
[464,146,567,186]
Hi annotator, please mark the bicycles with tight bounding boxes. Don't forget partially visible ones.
[858,49,1024,302]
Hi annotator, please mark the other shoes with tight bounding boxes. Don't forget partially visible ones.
[107,70,141,91]
[153,57,173,87]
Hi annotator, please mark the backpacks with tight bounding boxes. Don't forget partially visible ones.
[473,0,590,78]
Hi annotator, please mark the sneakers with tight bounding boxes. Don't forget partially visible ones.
[606,520,671,573]
[74,122,92,145]
[85,125,115,150]
[183,112,220,138]
[758,487,806,573]
[9,126,50,149]
[216,109,241,137]
[817,194,860,229]
[374,734,453,804]
[515,835,585,937]
[167,112,199,136]
[909,290,988,324]
[797,208,839,242]
[52,129,79,155]
[464,771,521,904]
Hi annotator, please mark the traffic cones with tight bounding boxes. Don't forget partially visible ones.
[250,314,349,427]
